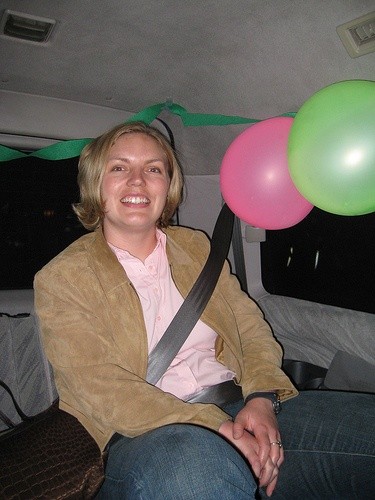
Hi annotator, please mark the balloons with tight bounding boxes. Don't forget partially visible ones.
[219,115,315,232]
[286,81,375,216]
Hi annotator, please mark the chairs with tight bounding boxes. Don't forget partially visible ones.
[0,291,63,435]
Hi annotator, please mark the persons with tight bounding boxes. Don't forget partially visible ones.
[34,122,375,500]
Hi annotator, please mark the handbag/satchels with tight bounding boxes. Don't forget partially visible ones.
[1,400,105,499]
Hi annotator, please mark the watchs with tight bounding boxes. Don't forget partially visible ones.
[244,392,281,418]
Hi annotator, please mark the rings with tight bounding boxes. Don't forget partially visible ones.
[270,440,283,448]
